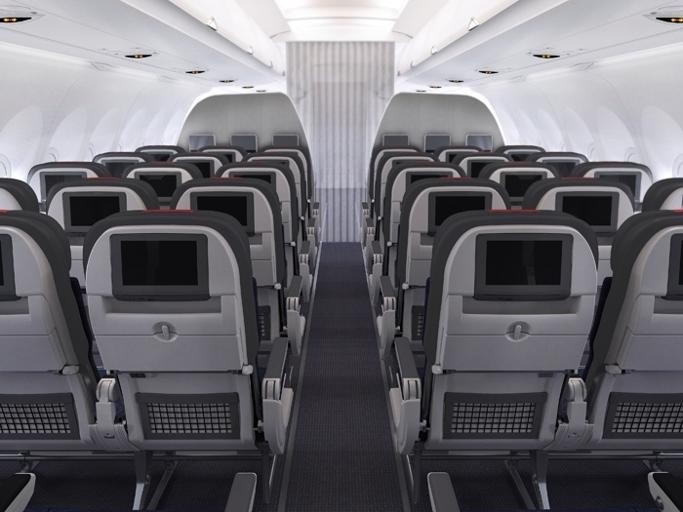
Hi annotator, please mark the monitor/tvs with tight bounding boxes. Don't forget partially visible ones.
[556,192,620,237]
[428,192,491,237]
[475,234,572,302]
[423,134,452,153]
[110,233,209,300]
[395,151,579,188]
[189,134,216,152]
[272,134,299,147]
[661,233,682,301]
[99,148,288,205]
[40,172,87,206]
[62,192,126,234]
[229,134,258,153]
[190,191,255,237]
[594,172,642,203]
[1,234,21,302]
[465,134,494,152]
[499,172,546,206]
[382,133,409,146]
